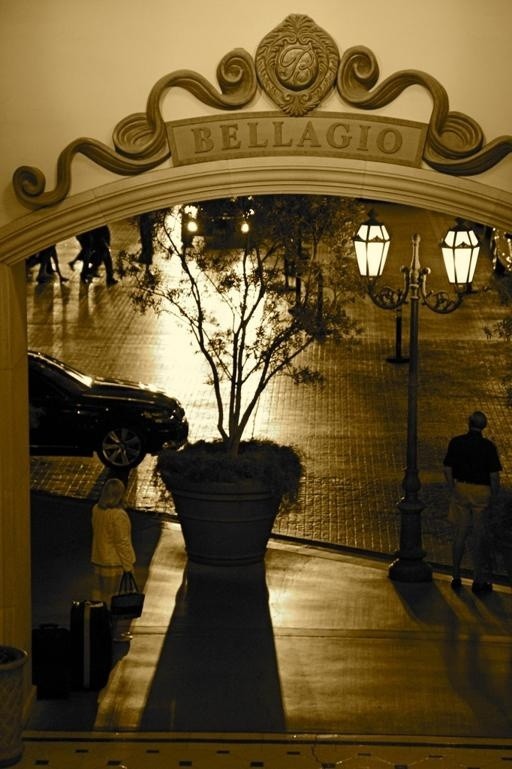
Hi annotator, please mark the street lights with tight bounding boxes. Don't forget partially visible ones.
[344,208,485,581]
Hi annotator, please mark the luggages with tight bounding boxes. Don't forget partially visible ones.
[68,600,113,692]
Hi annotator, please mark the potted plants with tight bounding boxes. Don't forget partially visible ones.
[109,185,373,569]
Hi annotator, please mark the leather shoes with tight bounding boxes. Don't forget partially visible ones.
[472,582,492,593]
[451,578,462,589]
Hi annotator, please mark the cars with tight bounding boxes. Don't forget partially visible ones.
[27,344,191,475]
[179,195,269,254]
[489,229,511,279]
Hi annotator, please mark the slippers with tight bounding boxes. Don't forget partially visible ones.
[121,631,133,639]
[113,634,129,641]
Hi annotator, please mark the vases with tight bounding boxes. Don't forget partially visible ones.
[1,643,30,766]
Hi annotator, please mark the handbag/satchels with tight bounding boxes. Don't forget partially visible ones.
[109,571,146,621]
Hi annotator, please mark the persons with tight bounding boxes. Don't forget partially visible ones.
[132,210,159,280]
[441,411,502,593]
[88,478,135,644]
[20,225,119,291]
